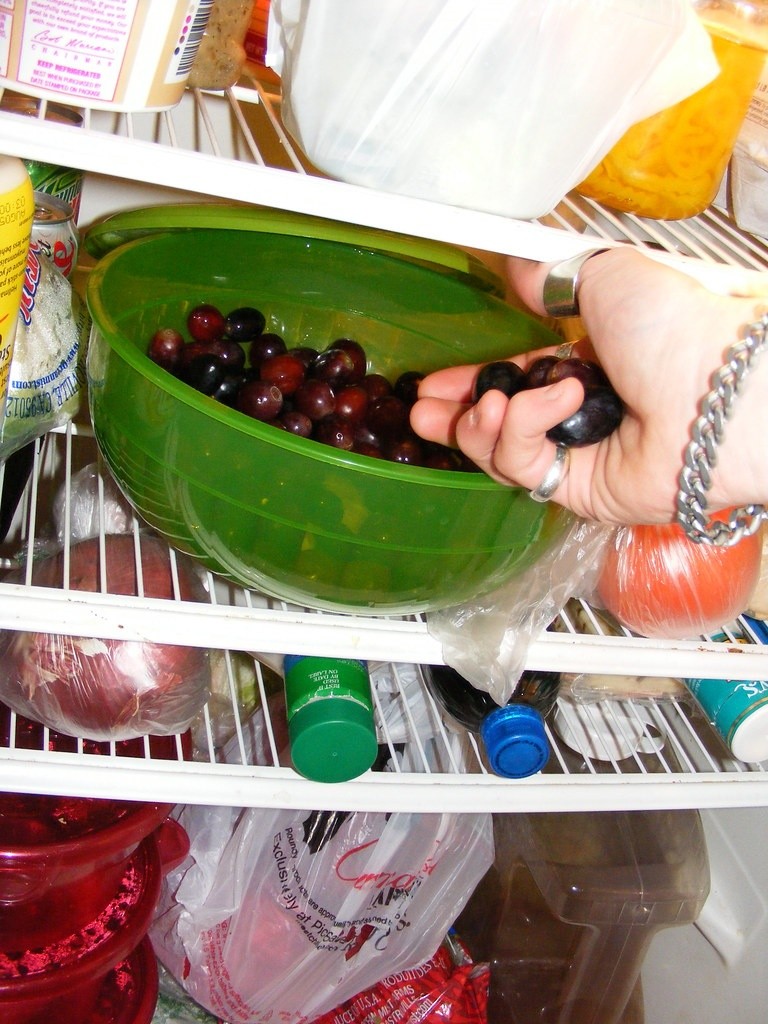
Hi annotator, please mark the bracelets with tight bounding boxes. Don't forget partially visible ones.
[673,314,767,549]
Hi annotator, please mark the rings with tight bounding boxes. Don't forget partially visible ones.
[546,244,610,321]
[552,338,582,380]
[526,441,571,504]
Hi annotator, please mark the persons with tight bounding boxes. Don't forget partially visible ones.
[410,244,767,525]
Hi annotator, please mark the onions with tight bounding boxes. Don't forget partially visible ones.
[0,533,213,731]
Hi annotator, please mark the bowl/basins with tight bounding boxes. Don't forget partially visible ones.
[85,232,576,615]
[0,791,191,1024]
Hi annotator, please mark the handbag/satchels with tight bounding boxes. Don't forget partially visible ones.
[139,663,496,1024]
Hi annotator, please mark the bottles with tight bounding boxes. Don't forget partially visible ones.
[282,654,379,783]
[419,662,560,779]
[0,153,35,436]
[448,698,712,1024]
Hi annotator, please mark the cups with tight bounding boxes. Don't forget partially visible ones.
[573,0,768,221]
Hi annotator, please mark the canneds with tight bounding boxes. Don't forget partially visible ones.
[0,98,84,226]
[4,192,78,280]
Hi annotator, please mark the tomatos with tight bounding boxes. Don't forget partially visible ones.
[597,506,762,635]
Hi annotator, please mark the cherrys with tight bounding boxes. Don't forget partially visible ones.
[148,304,627,481]
